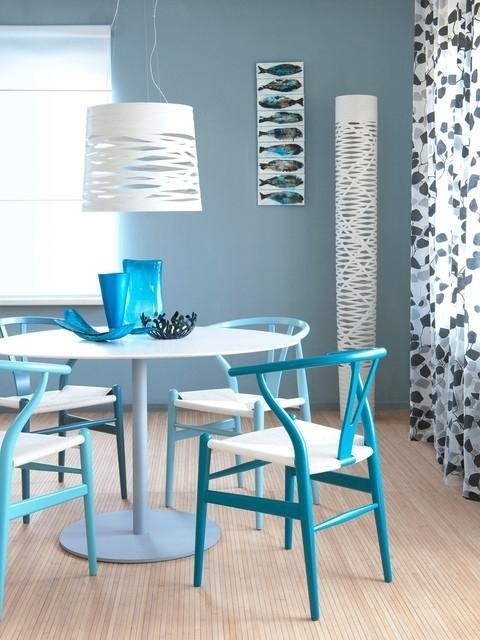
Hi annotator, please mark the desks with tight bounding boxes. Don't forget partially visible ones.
[4,325,299,565]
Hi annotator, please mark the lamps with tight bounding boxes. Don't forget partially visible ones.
[80,0,204,214]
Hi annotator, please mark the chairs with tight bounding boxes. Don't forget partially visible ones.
[1,316,128,524]
[165,315,319,531]
[1,358,98,615]
[192,348,391,621]
